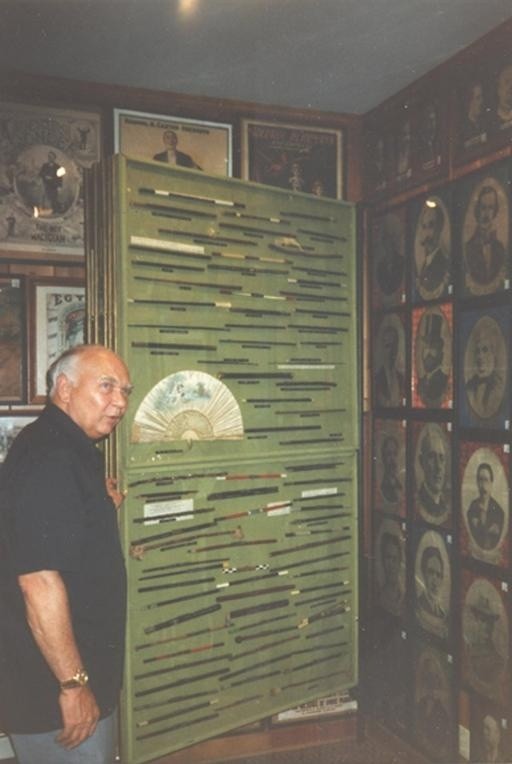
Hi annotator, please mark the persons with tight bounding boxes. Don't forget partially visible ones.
[38,151,65,215]
[374,186,508,764]
[0,344,133,764]
[154,130,195,169]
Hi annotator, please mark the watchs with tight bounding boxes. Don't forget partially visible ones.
[57,670,91,694]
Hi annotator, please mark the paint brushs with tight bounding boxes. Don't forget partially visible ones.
[125,188,356,740]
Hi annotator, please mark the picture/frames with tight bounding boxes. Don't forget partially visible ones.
[0,96,105,254]
[242,119,342,202]
[1,409,43,465]
[0,273,28,404]
[29,276,86,404]
[114,109,232,179]
